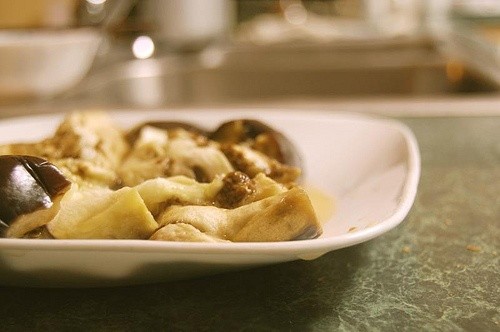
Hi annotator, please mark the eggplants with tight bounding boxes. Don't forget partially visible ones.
[0,153,72,239]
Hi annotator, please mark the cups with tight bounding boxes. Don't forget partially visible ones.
[161,0,237,51]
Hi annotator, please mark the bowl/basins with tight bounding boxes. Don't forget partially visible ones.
[0,30,99,100]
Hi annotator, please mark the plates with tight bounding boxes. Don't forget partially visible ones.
[0,111,421,285]
